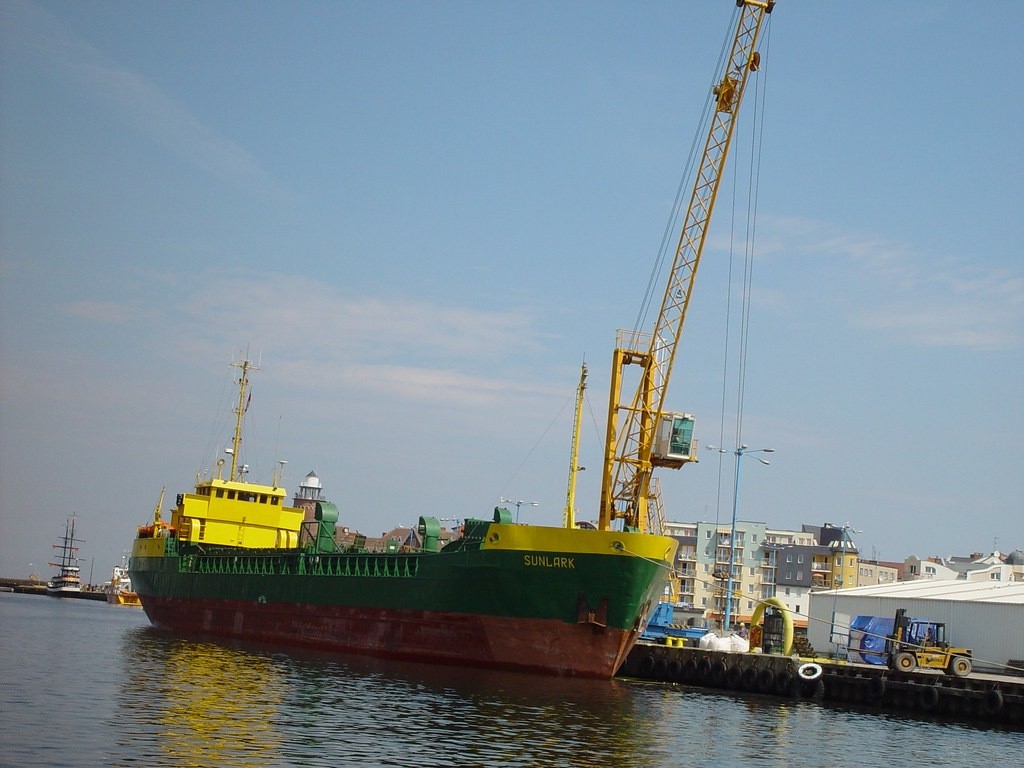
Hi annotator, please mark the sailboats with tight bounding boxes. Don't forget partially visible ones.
[46,511,87,598]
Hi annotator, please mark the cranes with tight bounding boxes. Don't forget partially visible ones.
[597,1,776,534]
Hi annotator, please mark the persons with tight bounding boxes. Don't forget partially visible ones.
[737,622,748,640]
[920,628,933,646]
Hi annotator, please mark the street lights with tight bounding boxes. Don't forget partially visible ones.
[704,444,775,632]
[500,496,538,524]
[759,543,794,598]
[831,522,863,589]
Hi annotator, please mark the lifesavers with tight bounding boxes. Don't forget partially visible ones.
[696,658,712,683]
[867,678,885,699]
[682,659,697,684]
[835,686,856,706]
[794,678,825,703]
[666,659,682,678]
[651,659,668,680]
[710,660,727,684]
[797,662,825,684]
[727,665,742,688]
[758,669,774,693]
[983,690,1004,714]
[774,670,794,695]
[742,664,760,692]
[758,664,769,677]
[916,686,938,710]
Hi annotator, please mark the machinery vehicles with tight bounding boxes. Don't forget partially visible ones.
[884,608,973,677]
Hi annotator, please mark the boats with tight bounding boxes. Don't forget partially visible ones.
[124,346,680,680]
[105,547,141,606]
